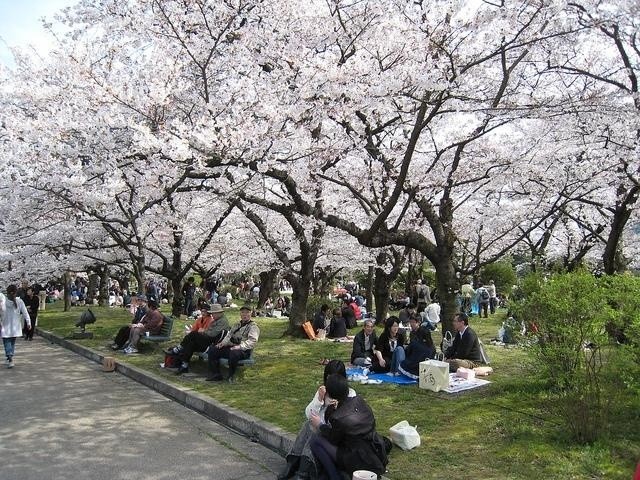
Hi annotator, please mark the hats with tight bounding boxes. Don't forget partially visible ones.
[138,295,148,301]
[200,304,210,310]
[240,306,252,311]
[207,304,224,313]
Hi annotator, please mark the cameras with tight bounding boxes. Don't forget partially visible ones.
[230,336,242,344]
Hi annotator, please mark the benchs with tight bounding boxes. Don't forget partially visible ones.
[140,315,174,352]
[191,348,255,367]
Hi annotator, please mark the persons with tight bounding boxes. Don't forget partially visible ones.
[0,285,31,368]
[312,293,364,339]
[109,297,163,353]
[70,276,88,306]
[33,281,63,311]
[145,280,167,299]
[16,280,39,341]
[182,277,227,317]
[240,280,261,299]
[280,281,285,291]
[389,279,441,332]
[109,285,138,307]
[352,310,480,379]
[277,359,388,480]
[265,295,292,316]
[499,285,531,344]
[462,277,496,318]
[164,304,260,381]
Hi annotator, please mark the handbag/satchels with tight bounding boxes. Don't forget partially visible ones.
[442,337,454,350]
[230,335,242,344]
[303,321,315,340]
[419,359,448,392]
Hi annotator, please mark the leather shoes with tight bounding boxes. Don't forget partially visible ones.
[161,348,223,381]
[277,464,309,480]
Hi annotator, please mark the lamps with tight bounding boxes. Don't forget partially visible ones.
[73,308,96,339]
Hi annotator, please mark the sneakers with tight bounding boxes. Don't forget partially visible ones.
[319,358,332,365]
[108,343,139,353]
[7,359,15,369]
[347,374,383,385]
